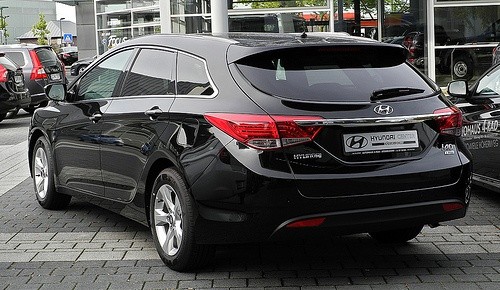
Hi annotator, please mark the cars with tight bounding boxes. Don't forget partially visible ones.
[70,53,107,75]
[56,45,81,66]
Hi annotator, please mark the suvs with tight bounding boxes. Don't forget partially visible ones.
[442,59,500,193]
[25,30,475,272]
[374,24,449,58]
[0,50,30,123]
[439,16,500,80]
[0,42,69,113]
